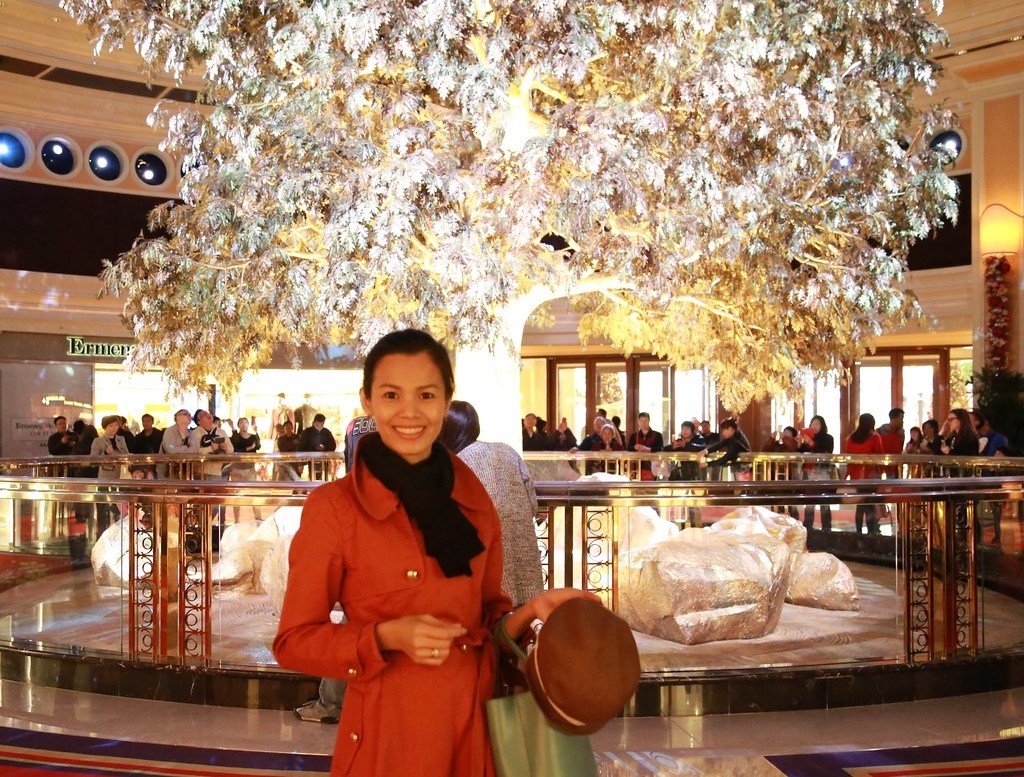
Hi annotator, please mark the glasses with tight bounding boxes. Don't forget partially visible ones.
[948,417,959,423]
[178,413,192,417]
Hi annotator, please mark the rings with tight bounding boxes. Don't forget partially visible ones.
[433,648,439,657]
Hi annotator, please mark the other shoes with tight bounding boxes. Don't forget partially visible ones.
[990,535,1002,540]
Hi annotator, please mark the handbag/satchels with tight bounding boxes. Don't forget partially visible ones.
[807,467,839,482]
[485,613,597,777]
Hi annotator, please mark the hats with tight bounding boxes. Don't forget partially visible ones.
[73,421,86,432]
[526,598,640,737]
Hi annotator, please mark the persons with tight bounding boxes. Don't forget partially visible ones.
[274,421,299,482]
[875,408,905,478]
[905,419,944,455]
[626,411,665,452]
[521,408,578,480]
[293,417,379,724]
[932,408,980,544]
[49,409,262,542]
[972,410,1009,542]
[440,400,543,606]
[272,328,601,777]
[763,426,800,519]
[269,394,316,438]
[699,417,753,481]
[799,414,834,531]
[298,414,337,481]
[847,414,883,534]
[663,418,719,480]
[577,409,626,481]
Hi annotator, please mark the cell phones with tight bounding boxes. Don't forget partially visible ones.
[941,440,946,447]
[700,457,707,464]
[777,425,782,434]
[800,427,814,439]
